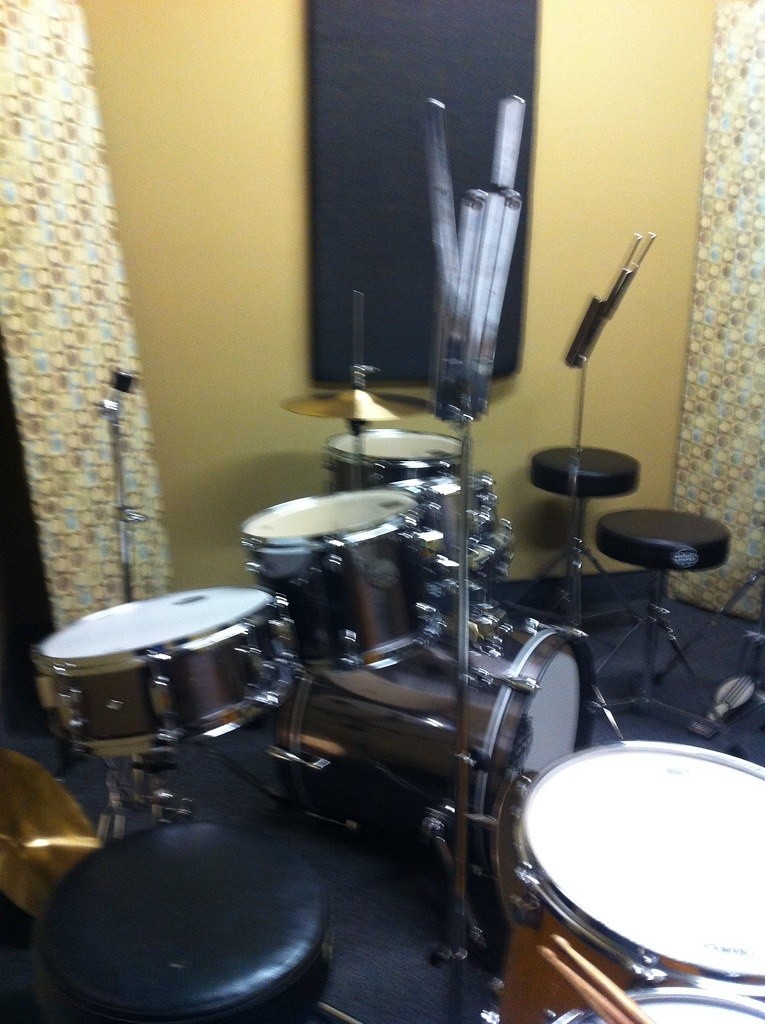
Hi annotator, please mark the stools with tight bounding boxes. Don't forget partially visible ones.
[590,509,735,733]
[527,444,641,622]
[43,819,358,1024]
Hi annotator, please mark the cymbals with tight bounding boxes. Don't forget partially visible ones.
[278,385,434,425]
[0,745,102,920]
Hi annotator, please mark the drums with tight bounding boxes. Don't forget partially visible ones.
[28,583,296,759]
[494,733,765,1024]
[271,598,601,887]
[236,487,446,677]
[323,426,466,492]
[375,468,502,599]
[544,985,765,1024]
